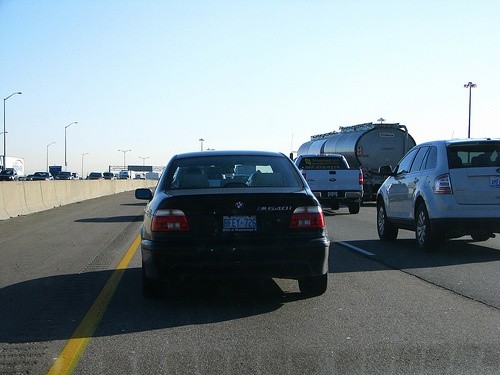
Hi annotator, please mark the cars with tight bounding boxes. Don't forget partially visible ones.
[87,172,102,180]
[30,172,54,181]
[375,138,500,249]
[103,171,145,179]
[56,172,74,180]
[0,168,17,181]
[135,150,330,297]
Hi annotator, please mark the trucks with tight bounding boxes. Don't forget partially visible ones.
[49,165,62,180]
[0,155,25,180]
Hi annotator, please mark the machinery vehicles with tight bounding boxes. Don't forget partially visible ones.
[296,122,417,206]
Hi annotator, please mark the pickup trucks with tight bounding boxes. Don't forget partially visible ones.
[293,154,364,213]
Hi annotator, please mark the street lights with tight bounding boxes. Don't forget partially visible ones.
[464,82,477,138]
[3,92,22,156]
[199,138,204,151]
[82,153,88,179]
[65,122,78,166]
[377,117,385,124]
[138,156,150,166]
[47,142,56,172]
[118,149,131,170]
